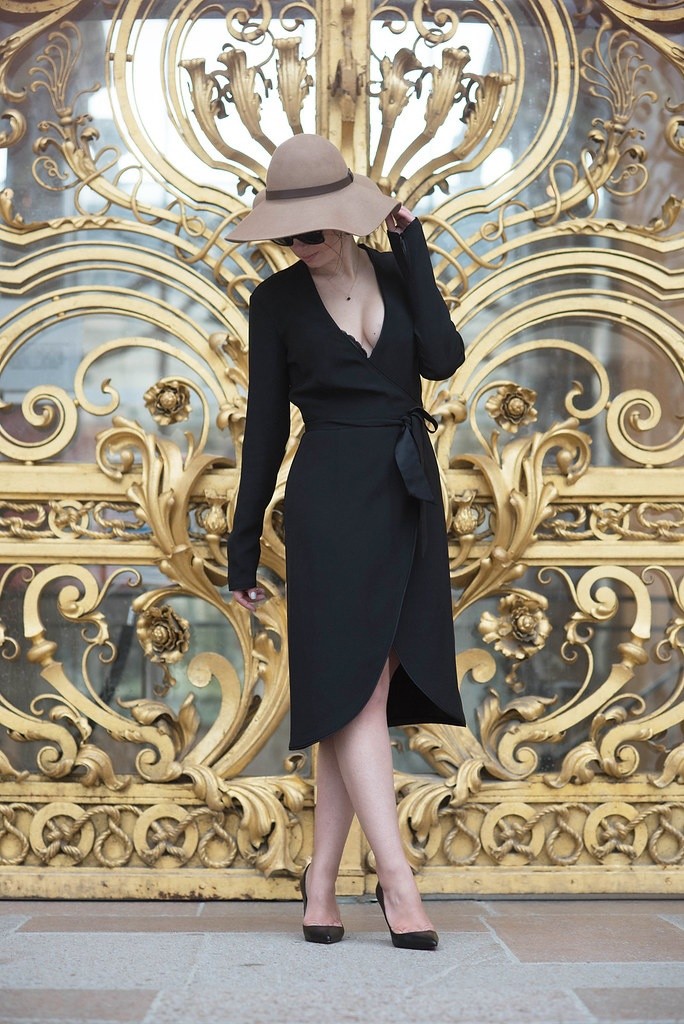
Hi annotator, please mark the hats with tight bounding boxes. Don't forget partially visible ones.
[224,134,402,243]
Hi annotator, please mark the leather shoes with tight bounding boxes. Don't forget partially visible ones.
[300,863,344,943]
[376,881,439,950]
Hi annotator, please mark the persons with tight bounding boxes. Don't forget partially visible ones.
[223,133,466,950]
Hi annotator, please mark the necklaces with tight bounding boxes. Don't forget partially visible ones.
[317,247,359,304]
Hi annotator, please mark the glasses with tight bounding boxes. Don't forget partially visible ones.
[271,231,325,246]
